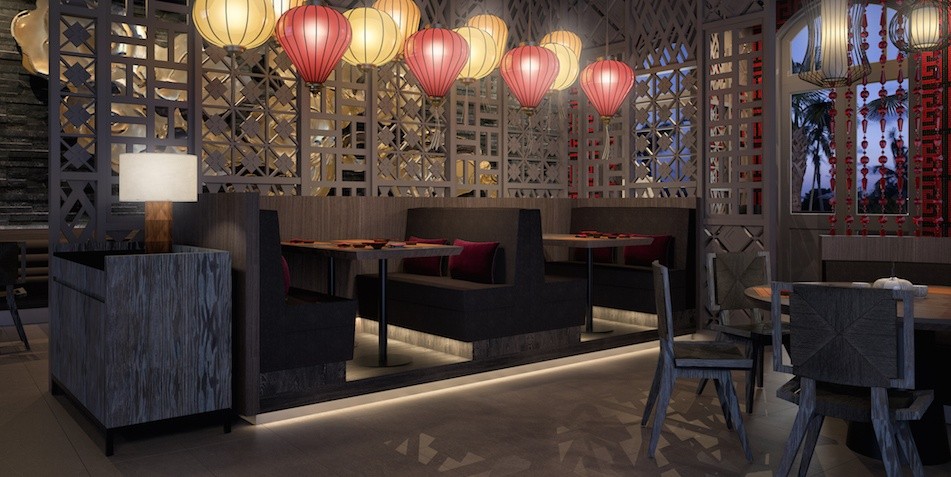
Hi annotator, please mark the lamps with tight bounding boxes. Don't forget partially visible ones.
[888,0,951,52]
[119,152,197,253]
[798,0,873,88]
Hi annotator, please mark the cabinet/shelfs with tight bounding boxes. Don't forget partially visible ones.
[48,242,234,457]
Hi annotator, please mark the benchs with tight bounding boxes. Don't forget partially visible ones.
[355,207,587,360]
[170,192,356,415]
[545,207,696,328]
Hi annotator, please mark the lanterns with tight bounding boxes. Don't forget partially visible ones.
[798,0,872,88]
[889,0,951,53]
[273,0,582,116]
[192,0,276,54]
[578,55,635,121]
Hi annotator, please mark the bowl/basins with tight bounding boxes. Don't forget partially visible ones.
[370,243,385,249]
[913,285,928,296]
[853,282,871,288]
[374,238,390,245]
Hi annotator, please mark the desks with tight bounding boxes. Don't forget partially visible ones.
[283,239,464,367]
[542,233,655,333]
[745,284,951,464]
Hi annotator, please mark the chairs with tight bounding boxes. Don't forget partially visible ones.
[694,250,791,415]
[640,259,756,467]
[0,241,31,351]
[770,281,937,477]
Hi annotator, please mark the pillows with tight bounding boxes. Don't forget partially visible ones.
[399,237,449,276]
[570,230,672,265]
[447,239,501,283]
